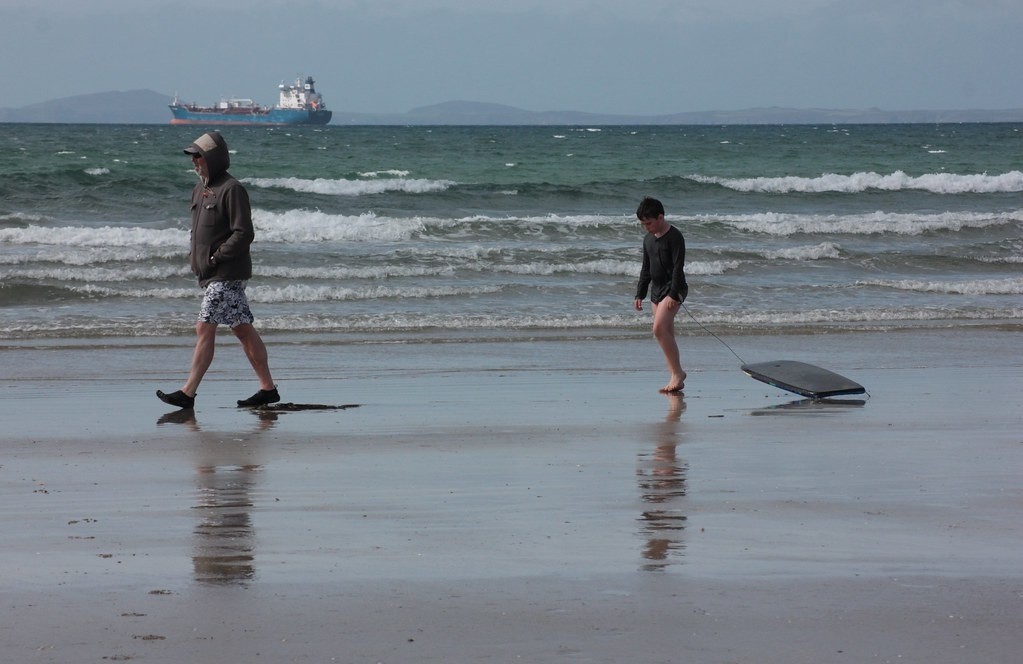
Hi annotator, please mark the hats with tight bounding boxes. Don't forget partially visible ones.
[183,147,199,155]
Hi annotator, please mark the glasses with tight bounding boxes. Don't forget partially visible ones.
[192,153,202,159]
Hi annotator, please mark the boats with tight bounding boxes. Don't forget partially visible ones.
[167,73,332,126]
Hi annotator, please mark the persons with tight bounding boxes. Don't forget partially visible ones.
[633,198,689,391]
[156,132,281,409]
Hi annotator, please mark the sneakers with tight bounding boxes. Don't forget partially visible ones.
[156,390,197,409]
[236,386,280,406]
